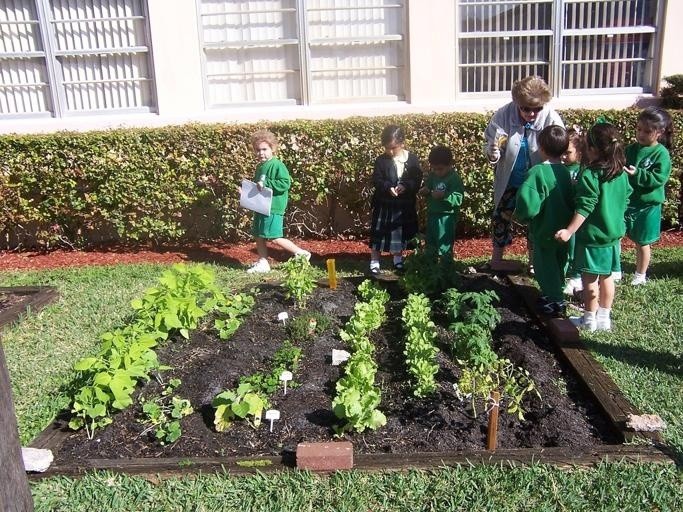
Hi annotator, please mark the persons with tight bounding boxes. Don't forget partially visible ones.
[478,75,567,277]
[368,124,423,275]
[245,129,311,275]
[559,123,588,296]
[553,122,634,332]
[512,124,575,318]
[623,106,675,286]
[418,145,465,256]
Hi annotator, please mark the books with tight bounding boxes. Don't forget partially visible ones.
[239,177,272,217]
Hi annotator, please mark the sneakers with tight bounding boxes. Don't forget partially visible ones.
[393,256,403,269]
[370,261,380,274]
[248,261,271,274]
[536,272,646,332]
[294,249,311,262]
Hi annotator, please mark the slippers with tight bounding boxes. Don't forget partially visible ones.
[478,262,492,271]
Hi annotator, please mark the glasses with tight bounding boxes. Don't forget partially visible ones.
[520,106,543,113]
